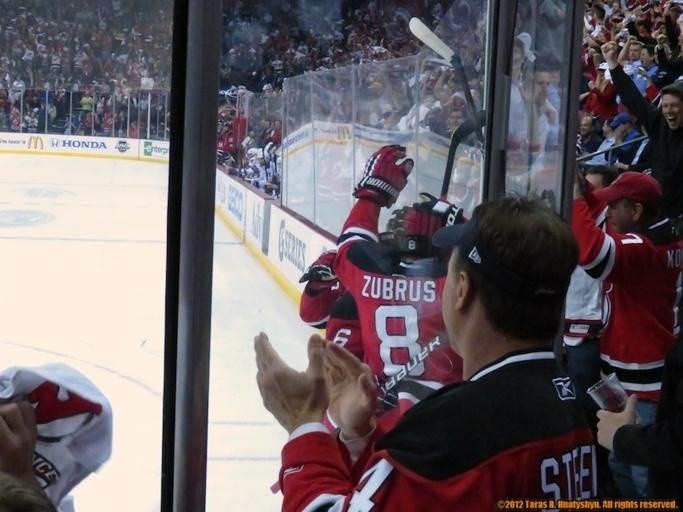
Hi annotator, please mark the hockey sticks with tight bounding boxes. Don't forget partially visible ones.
[441,110,486,200]
[409,17,484,160]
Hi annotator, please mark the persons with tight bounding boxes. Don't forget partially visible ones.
[1,0,683,512]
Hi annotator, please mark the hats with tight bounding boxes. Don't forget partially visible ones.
[592,171,663,205]
[609,112,632,127]
[596,62,607,70]
[432,200,568,302]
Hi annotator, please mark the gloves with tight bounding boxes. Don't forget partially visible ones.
[298,250,340,295]
[388,191,463,234]
[353,144,414,209]
